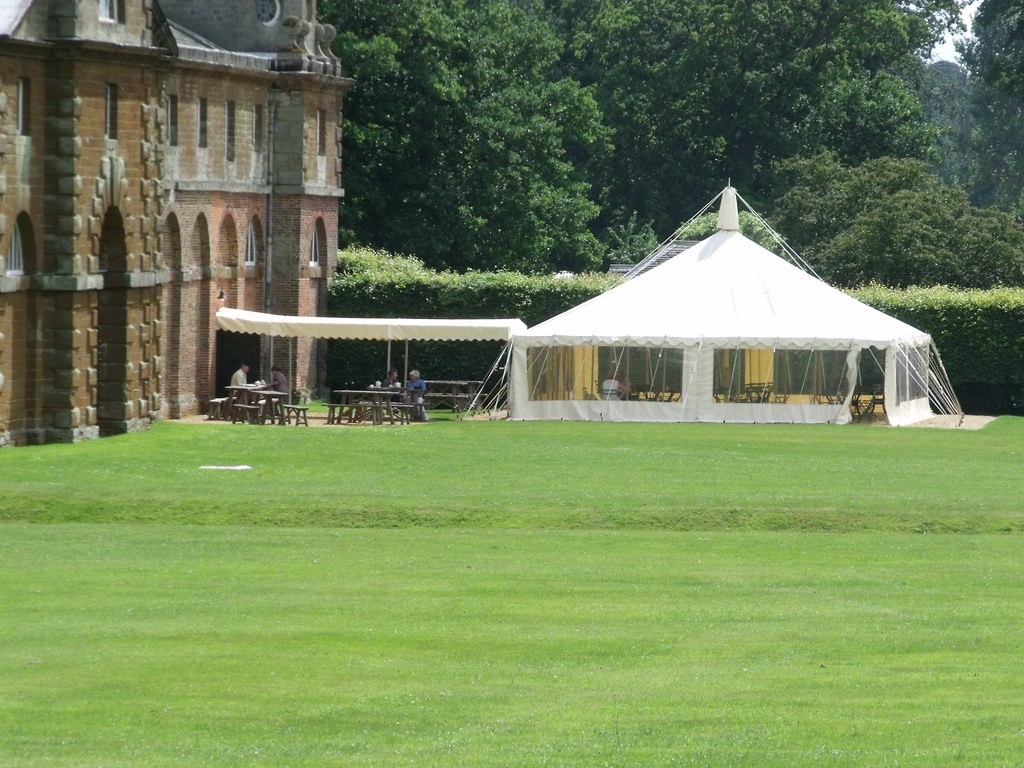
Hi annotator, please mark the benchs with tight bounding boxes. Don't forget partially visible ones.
[208,380,487,426]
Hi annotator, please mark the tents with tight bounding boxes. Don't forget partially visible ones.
[214,306,527,423]
[455,181,966,423]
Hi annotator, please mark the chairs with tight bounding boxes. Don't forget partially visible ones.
[581,382,887,424]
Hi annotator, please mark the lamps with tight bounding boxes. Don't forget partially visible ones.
[217,284,228,301]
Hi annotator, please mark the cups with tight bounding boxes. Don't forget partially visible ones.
[256,381,260,386]
[370,385,374,388]
[389,385,393,388]
[261,380,266,385]
[376,381,381,388]
[396,382,401,388]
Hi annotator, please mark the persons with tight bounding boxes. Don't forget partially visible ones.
[262,363,289,409]
[381,368,402,402]
[615,368,632,401]
[229,362,251,405]
[407,370,430,421]
[601,368,626,400]
